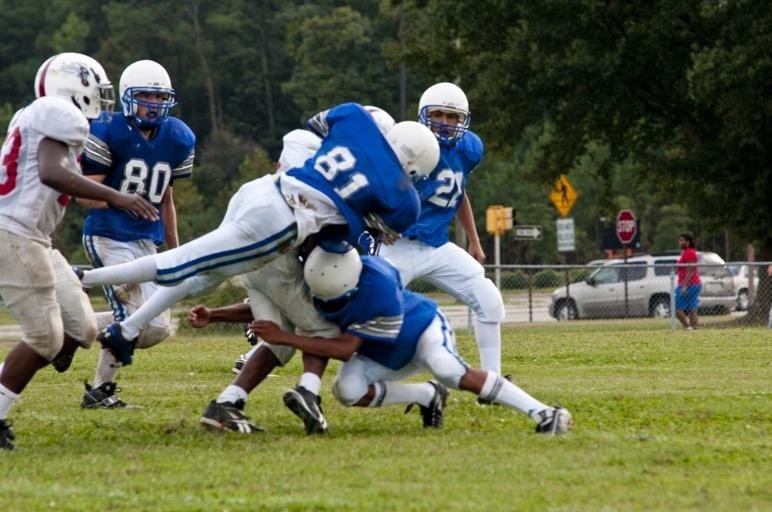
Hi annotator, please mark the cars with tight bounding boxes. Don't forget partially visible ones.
[724,262,760,311]
[574,258,606,283]
[547,250,738,322]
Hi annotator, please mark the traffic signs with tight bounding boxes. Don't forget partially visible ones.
[516,226,542,240]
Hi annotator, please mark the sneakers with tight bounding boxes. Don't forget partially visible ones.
[0,266,139,451]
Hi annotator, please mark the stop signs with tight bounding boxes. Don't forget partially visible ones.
[616,209,636,244]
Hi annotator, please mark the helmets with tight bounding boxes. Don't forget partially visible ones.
[362,105,396,135]
[418,82,472,145]
[34,52,115,123]
[119,59,177,127]
[384,120,440,186]
[303,241,361,297]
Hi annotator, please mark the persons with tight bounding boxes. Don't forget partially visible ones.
[0,49,161,452]
[50,81,573,437]
[673,233,704,329]
[74,58,197,410]
